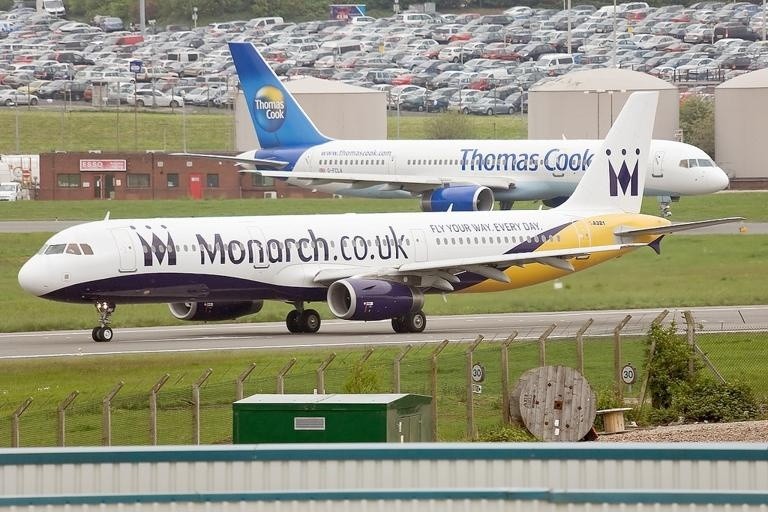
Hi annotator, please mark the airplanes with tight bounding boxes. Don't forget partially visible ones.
[16,89,749,342]
[169,41,731,218]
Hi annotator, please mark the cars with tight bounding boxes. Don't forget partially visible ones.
[1,0,768,114]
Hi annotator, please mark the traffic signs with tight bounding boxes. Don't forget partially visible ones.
[472,364,483,381]
[622,365,634,384]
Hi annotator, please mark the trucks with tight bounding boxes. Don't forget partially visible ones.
[0,181,30,202]
